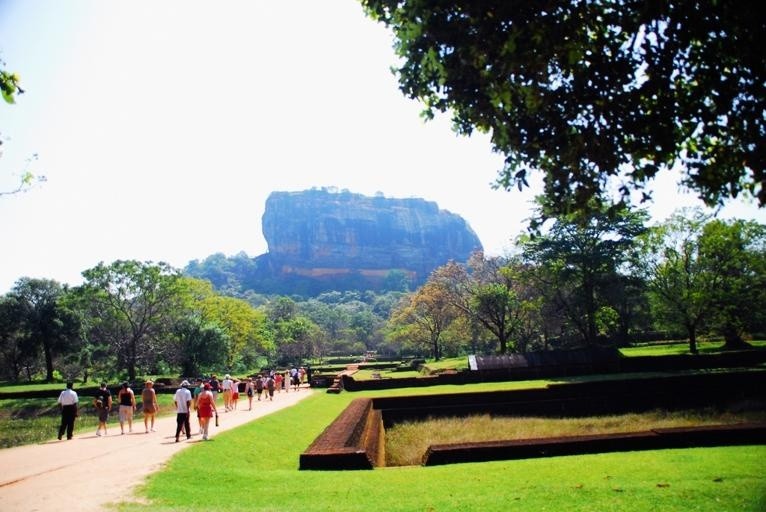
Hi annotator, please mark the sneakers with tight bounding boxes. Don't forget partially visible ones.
[59,432,62,440]
[146,428,156,433]
[199,429,208,441]
[68,436,71,439]
[176,432,190,442]
[122,429,132,435]
[96,431,108,436]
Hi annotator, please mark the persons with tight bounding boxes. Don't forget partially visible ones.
[170,364,307,441]
[116,381,137,434]
[142,379,160,433]
[93,381,112,437]
[56,380,80,439]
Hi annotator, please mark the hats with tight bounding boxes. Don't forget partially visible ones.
[182,381,190,386]
[205,383,212,388]
